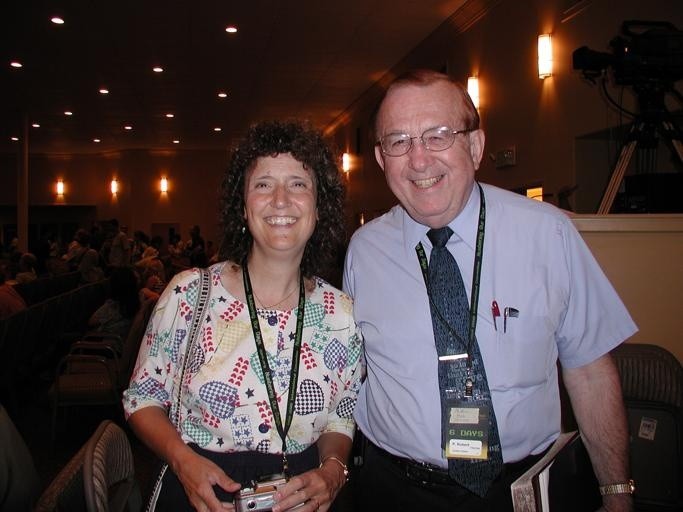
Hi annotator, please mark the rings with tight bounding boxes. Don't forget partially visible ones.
[308,496,320,510]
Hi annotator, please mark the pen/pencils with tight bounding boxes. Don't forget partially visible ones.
[491,300,509,333]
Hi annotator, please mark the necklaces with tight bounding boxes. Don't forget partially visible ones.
[249,268,301,310]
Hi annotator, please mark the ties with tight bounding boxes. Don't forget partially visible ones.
[424,229,509,498]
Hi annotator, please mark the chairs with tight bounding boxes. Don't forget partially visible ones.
[26,257,683,512]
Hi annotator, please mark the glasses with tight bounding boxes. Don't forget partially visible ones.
[376,127,473,157]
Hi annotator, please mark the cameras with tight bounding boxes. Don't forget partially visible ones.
[235,481,305,512]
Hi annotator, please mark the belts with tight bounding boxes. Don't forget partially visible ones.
[378,455,524,488]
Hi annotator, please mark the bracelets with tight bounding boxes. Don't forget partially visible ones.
[598,477,640,498]
[317,454,350,488]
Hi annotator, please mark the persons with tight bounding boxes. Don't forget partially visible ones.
[121,117,366,512]
[340,67,641,512]
[0,216,226,512]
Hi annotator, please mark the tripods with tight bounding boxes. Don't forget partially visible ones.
[597,124,683,214]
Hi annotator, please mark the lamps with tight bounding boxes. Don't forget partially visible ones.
[466,73,480,115]
[537,33,554,79]
[342,151,351,172]
